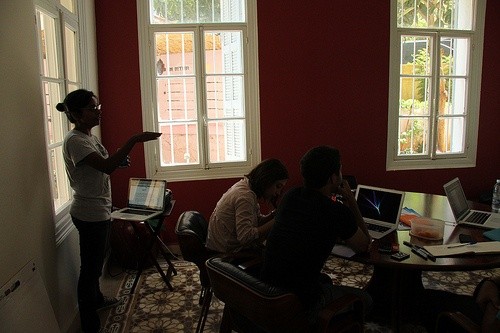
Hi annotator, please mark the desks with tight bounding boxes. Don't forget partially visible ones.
[331,192,500,333]
[131,200,177,292]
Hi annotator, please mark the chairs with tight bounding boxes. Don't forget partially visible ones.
[434,276,500,332]
[205,252,360,333]
[175,210,256,333]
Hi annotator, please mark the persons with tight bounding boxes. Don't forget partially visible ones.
[56,89,162,333]
[268,146,384,333]
[203,157,289,255]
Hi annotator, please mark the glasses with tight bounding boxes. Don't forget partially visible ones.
[80,103,102,112]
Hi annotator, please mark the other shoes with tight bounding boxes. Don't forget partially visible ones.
[97,295,122,311]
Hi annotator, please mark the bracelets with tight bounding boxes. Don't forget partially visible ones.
[271,210,275,215]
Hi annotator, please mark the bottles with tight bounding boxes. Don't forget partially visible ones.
[491,179,500,210]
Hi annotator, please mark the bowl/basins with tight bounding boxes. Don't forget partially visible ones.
[410,218,445,240]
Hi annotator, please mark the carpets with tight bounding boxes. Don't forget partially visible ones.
[102,244,500,333]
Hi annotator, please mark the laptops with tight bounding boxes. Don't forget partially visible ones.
[442,177,500,230]
[355,185,405,239]
[110,178,167,222]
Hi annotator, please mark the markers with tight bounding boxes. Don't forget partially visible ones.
[403,241,436,261]
